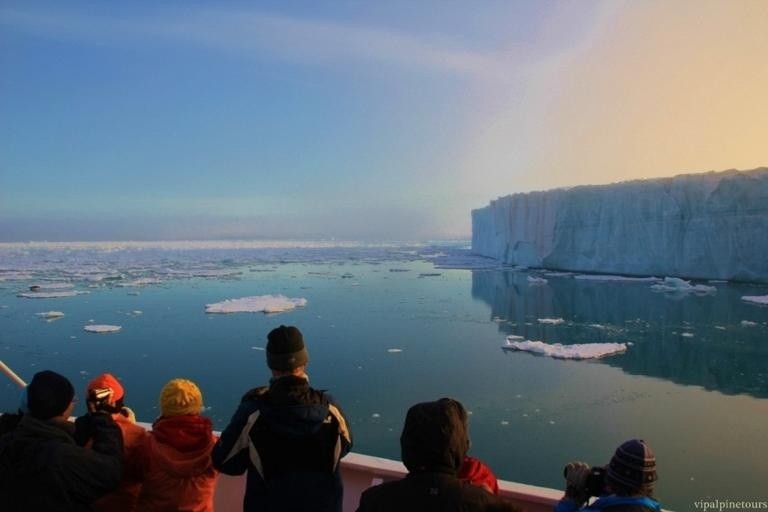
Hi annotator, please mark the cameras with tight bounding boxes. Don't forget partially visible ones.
[88,387,114,409]
[564,466,617,497]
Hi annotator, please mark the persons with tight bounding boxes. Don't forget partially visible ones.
[435,396,498,491]
[356,400,516,511]
[0,368,122,511]
[127,375,226,512]
[211,325,352,512]
[551,436,662,511]
[77,373,156,512]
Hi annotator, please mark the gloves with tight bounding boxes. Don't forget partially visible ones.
[565,462,592,507]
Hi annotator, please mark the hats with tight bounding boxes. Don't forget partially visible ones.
[161,379,202,416]
[606,439,658,488]
[88,374,124,403]
[28,371,74,418]
[266,326,307,370]
